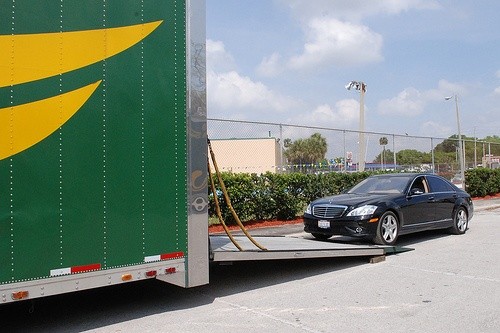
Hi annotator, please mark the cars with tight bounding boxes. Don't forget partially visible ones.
[444,95,466,184]
[302,172,475,246]
